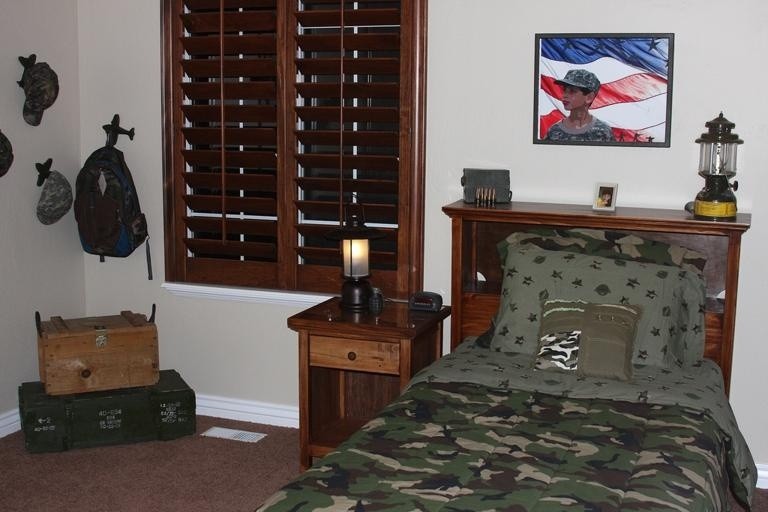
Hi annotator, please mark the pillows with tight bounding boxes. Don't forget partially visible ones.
[534,291,645,381]
[475,225,708,348]
[672,265,706,370]
[487,240,683,372]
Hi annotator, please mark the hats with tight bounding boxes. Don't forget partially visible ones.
[555,67,601,93]
[37,170,74,225]
[22,62,59,127]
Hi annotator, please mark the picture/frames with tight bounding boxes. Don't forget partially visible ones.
[592,182,618,211]
[531,28,678,151]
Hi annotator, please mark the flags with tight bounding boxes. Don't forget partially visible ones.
[538,37,669,144]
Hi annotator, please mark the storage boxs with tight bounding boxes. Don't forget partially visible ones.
[14,365,198,452]
[33,309,160,397]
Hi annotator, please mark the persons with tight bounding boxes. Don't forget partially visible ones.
[542,69,616,142]
[619,134,625,142]
[633,133,643,144]
[595,190,612,208]
[645,136,655,144]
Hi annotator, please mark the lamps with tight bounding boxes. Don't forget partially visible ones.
[329,198,381,314]
[682,110,750,220]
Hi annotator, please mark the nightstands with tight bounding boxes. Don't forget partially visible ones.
[288,294,445,473]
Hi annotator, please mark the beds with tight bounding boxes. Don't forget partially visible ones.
[246,194,761,512]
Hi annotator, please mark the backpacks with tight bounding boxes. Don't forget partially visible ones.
[73,145,147,262]
